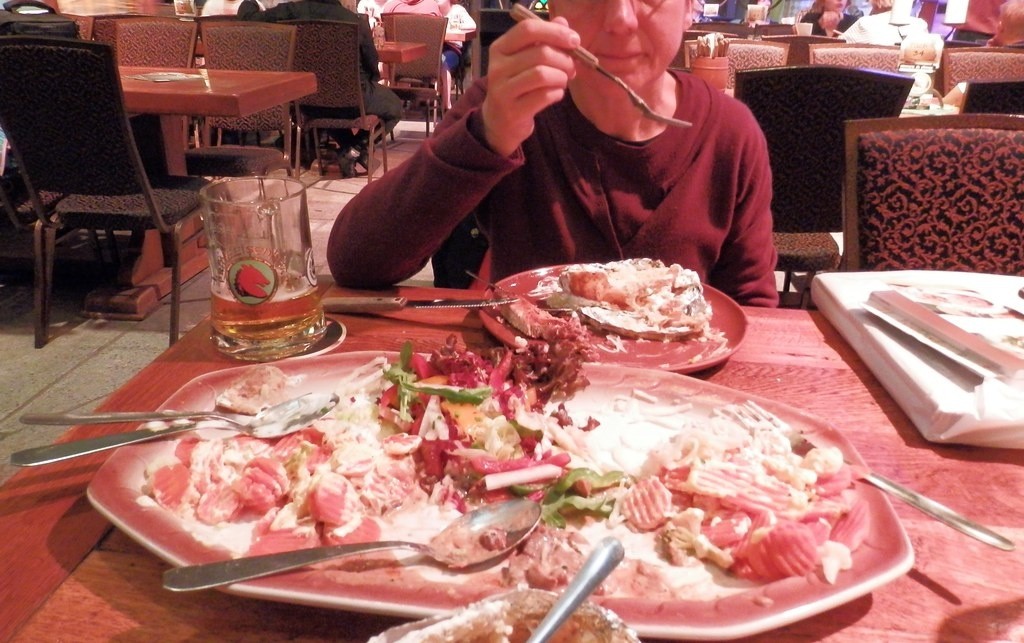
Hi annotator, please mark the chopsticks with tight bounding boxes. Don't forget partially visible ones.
[692,32,730,57]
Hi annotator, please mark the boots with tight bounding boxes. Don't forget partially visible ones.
[358,150,382,174]
[336,145,361,178]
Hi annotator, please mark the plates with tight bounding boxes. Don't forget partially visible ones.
[476,263,749,374]
[85,340,920,642]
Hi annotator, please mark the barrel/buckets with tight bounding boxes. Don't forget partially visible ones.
[691,54,728,96]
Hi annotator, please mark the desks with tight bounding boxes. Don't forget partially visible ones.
[0,273,1024,641]
[78,65,319,323]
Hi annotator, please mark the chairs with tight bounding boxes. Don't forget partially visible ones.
[51,1,1023,307]
[0,35,223,350]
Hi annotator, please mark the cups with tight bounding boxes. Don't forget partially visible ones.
[794,23,814,35]
[373,21,386,48]
[198,175,328,362]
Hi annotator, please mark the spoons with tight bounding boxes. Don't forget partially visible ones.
[463,270,577,314]
[507,3,694,130]
[164,497,545,593]
[18,395,339,440]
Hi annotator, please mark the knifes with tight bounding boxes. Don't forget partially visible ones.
[322,292,519,315]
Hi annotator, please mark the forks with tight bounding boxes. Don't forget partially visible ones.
[725,396,1014,551]
[10,390,313,469]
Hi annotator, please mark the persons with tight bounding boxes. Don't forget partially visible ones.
[320,2,782,311]
[202,1,267,25]
[256,2,406,177]
[433,1,477,117]
[943,1,1023,115]
[803,0,855,43]
[842,0,935,46]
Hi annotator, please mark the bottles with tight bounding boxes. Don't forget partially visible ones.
[193,36,206,67]
